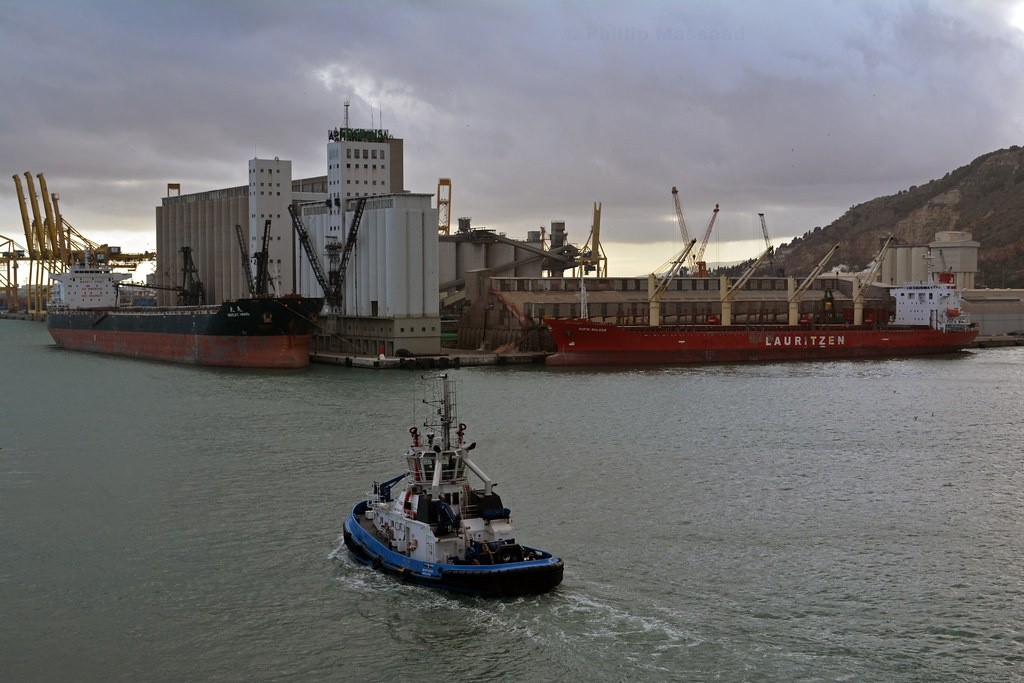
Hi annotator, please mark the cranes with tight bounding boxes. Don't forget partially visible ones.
[758,213,777,277]
[671,186,720,277]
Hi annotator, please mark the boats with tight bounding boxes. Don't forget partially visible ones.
[541,250,979,365]
[45,245,325,368]
[343,372,564,598]
